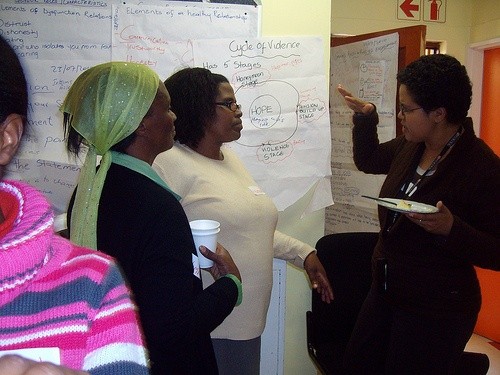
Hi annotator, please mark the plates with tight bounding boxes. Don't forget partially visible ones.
[375,197,439,214]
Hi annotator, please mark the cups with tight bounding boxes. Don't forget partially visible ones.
[191,227,221,269]
[189,219,221,234]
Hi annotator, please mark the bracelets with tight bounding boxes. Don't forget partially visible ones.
[222,273,242,307]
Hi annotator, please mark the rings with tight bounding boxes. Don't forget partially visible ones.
[417,220,421,224]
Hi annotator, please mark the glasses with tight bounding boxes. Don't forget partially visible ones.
[400,104,434,116]
[214,100,241,112]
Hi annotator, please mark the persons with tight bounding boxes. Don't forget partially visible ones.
[0,31,152,375]
[59,62,334,375]
[337,54,499,374]
